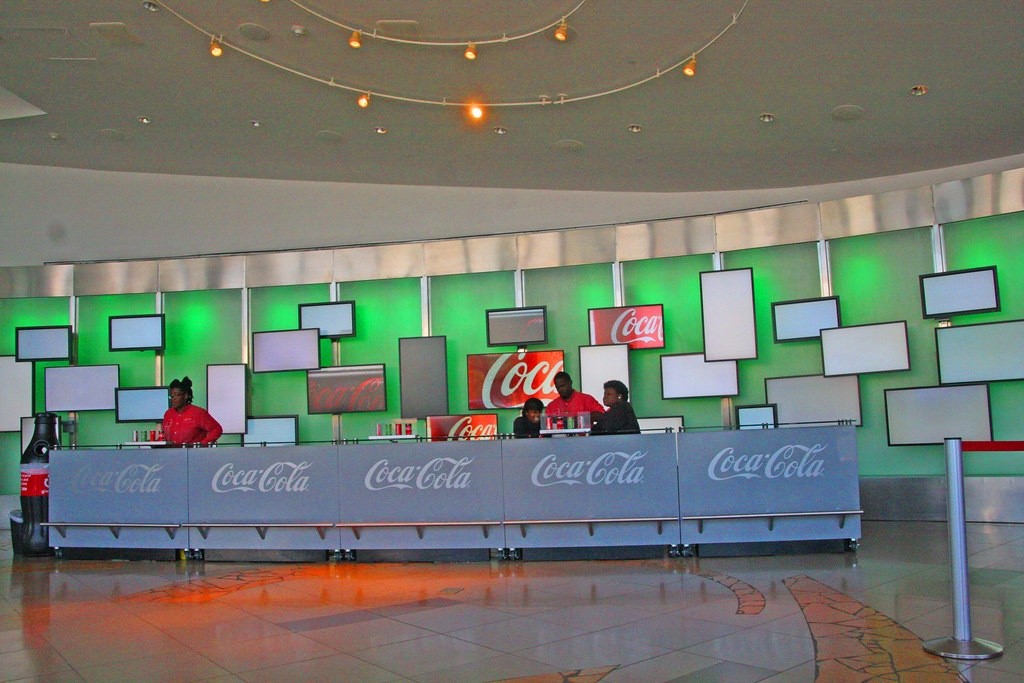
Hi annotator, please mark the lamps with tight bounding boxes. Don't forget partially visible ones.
[684,52,696,77]
[208,33,224,58]
[464,42,478,60]
[358,92,372,108]
[349,31,362,50]
[554,17,568,41]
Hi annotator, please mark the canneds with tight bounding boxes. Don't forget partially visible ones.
[158,430,165,441]
[556,416,564,429]
[385,423,392,435]
[396,423,402,435]
[566,417,574,428]
[376,424,383,435]
[150,430,156,441]
[405,423,412,435]
[545,416,553,430]
[132,430,138,442]
[141,430,147,441]
[577,415,585,428]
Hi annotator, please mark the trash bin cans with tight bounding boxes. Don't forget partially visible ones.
[8,509,24,555]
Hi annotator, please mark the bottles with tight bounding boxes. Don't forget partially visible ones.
[20,413,61,556]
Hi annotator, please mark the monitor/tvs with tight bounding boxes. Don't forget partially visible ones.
[0,265,1024,462]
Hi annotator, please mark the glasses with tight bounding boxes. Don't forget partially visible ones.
[168,393,185,400]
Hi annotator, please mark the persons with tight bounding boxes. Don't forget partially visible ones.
[590,380,641,436]
[161,376,223,448]
[546,371,605,436]
[514,398,544,437]
